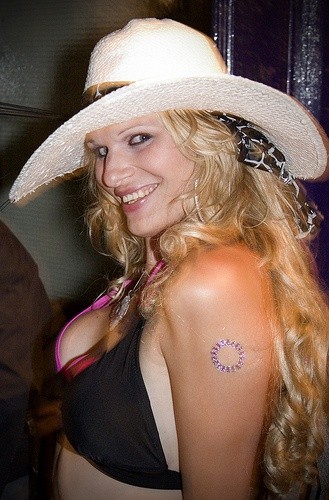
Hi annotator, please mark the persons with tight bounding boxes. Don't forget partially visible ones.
[9,18,329,500]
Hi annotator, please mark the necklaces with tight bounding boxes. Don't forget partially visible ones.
[109,269,148,332]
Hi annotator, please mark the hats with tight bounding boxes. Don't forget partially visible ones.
[9,18,329,207]
